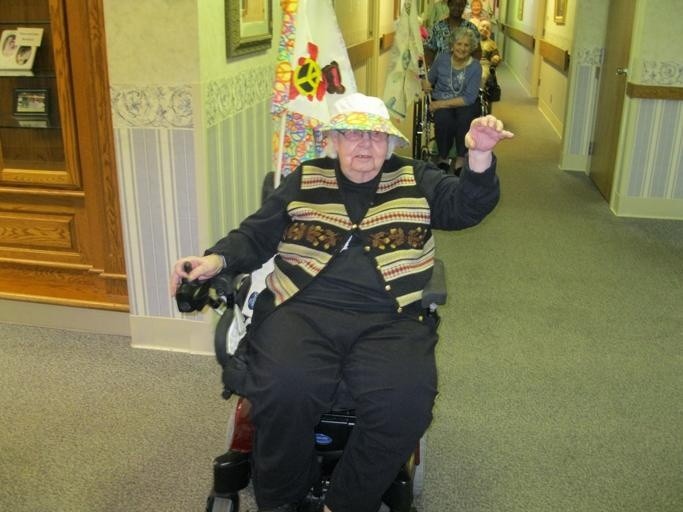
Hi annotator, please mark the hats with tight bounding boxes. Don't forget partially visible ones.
[319,92,410,149]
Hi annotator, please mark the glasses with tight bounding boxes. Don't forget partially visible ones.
[335,129,389,142]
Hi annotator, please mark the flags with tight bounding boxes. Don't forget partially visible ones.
[270,0,359,181]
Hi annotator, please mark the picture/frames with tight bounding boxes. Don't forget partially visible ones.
[553,0,568,24]
[12,87,51,117]
[224,0,274,59]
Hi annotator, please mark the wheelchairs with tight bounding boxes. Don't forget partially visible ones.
[176,171,446,512]
[412,56,496,159]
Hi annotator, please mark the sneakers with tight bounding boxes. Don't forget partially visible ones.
[437,162,464,177]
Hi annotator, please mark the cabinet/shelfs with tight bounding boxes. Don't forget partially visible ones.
[0,21,61,130]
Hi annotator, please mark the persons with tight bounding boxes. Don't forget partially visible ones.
[477,21,502,91]
[419,28,484,179]
[422,1,482,71]
[463,0,490,25]
[416,0,451,77]
[167,90,515,511]
[27,98,40,109]
[1,35,16,57]
